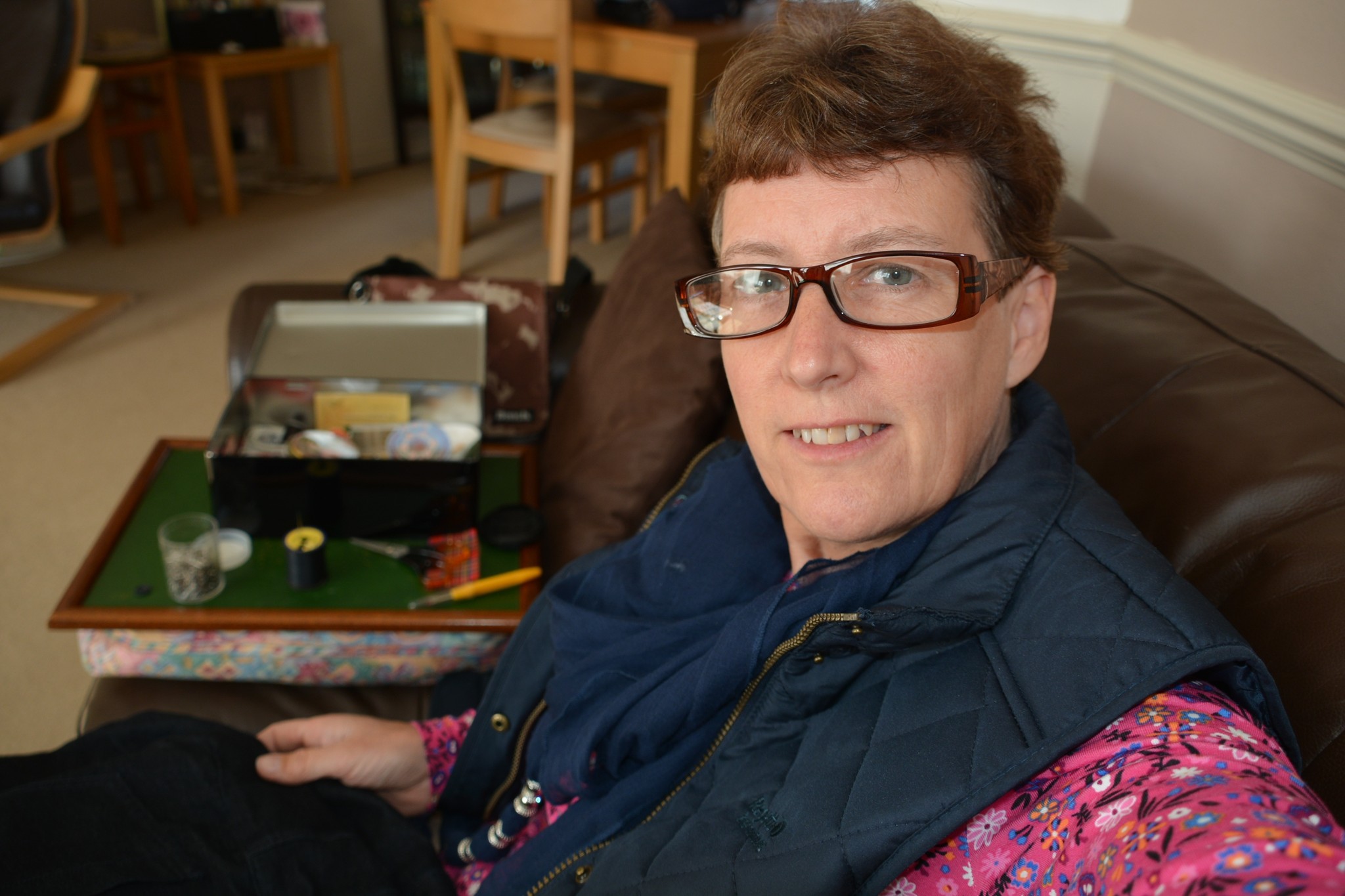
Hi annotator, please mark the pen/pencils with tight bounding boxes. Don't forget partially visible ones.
[408,566,544,615]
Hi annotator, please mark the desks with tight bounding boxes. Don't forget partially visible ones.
[492,20,748,241]
[166,43,350,217]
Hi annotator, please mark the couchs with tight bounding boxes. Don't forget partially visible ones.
[555,139,1345,896]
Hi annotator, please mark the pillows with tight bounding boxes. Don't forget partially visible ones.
[542,186,735,575]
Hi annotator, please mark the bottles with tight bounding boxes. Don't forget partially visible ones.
[158,510,225,604]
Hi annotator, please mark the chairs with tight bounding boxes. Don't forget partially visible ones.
[424,0,655,291]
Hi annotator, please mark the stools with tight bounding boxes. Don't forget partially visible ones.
[60,65,197,250]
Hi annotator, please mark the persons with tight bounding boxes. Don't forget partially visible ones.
[0,2,1343,894]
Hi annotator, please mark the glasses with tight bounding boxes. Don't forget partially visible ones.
[675,251,1041,339]
